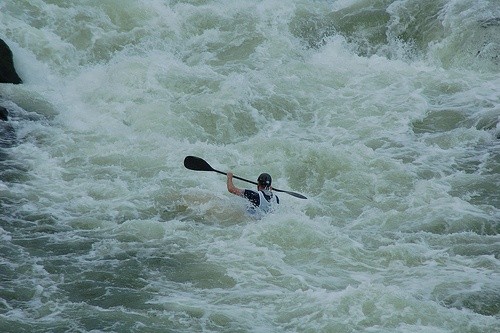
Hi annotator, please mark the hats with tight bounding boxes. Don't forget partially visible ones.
[257,172,272,186]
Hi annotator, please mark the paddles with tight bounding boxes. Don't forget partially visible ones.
[184,155,307,200]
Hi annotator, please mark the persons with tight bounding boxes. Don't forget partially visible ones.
[225,172,279,219]
